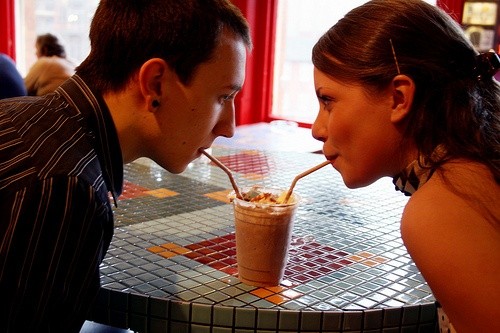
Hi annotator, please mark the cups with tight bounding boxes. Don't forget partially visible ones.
[229,185,301,287]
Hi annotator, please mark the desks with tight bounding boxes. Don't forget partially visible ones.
[87,123,456,331]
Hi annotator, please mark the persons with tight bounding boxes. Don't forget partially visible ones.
[24,32,74,94]
[3,48,24,100]
[3,1,255,325]
[313,1,494,330]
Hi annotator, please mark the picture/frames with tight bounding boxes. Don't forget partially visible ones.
[460,0,500,29]
